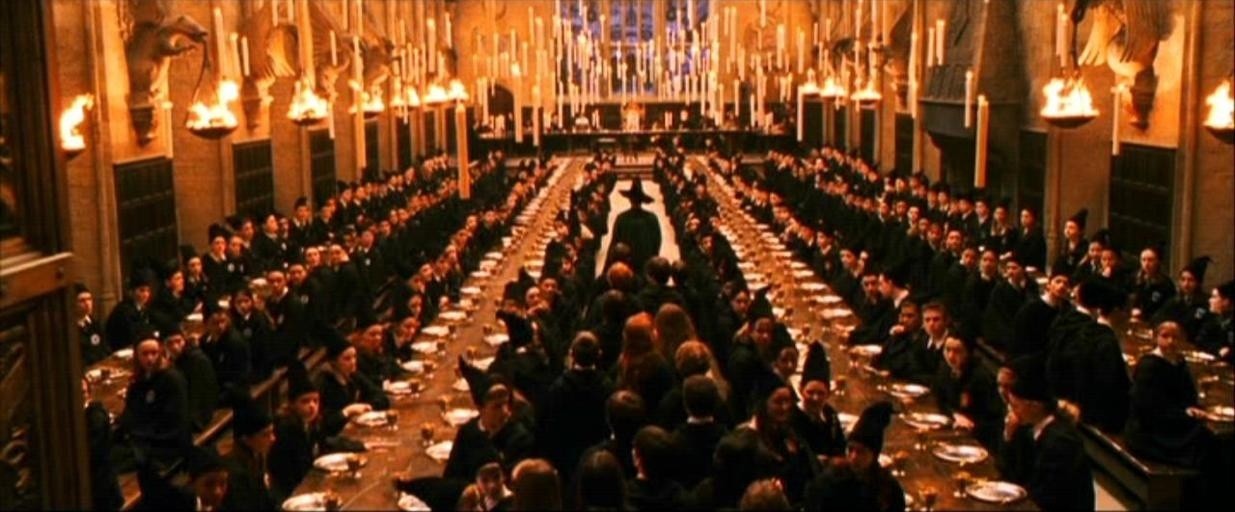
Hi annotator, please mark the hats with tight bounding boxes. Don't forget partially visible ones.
[68,140,1234,512]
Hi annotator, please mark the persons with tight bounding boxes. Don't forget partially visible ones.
[69,110,1235,511]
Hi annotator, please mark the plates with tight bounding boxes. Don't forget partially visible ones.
[974,244,1235,423]
[677,149,1026,512]
[78,154,595,512]
